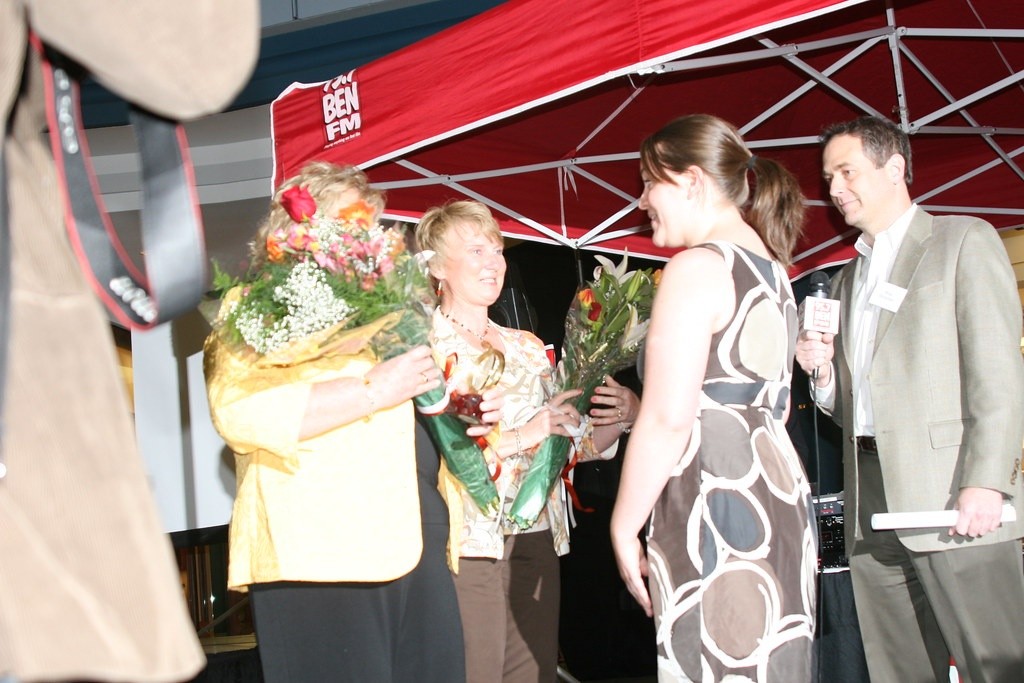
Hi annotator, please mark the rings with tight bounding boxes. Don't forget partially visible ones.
[420,373,428,383]
[616,407,621,417]
[570,414,575,419]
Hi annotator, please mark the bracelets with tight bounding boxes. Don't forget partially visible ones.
[514,429,524,456]
[617,422,632,433]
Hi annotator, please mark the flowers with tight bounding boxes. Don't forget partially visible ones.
[509,246,662,529]
[209,182,500,520]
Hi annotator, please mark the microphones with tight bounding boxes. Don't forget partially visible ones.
[796,270,841,378]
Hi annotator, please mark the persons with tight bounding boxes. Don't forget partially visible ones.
[417,202,639,683]
[612,115,818,681]
[0,1,260,683]
[795,117,1024,683]
[204,162,503,683]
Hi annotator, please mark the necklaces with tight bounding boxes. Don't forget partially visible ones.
[440,307,489,339]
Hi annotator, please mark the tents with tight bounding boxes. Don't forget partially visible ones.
[271,0,1023,286]
